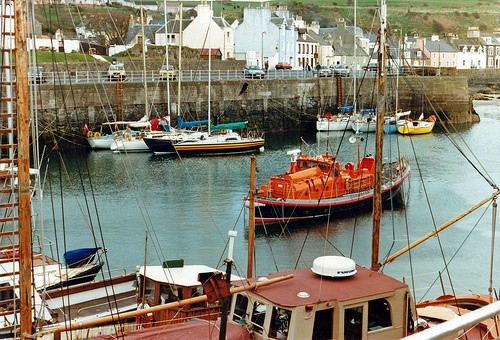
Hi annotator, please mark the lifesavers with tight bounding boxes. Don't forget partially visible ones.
[94,132,100,138]
[325,112,332,119]
[136,303,154,328]
[87,131,93,137]
[124,133,130,139]
[113,134,118,139]
[407,319,429,335]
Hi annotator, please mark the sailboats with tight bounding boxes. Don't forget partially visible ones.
[316,0,436,136]
[90,0,500,340]
[0,0,247,339]
[85,0,265,158]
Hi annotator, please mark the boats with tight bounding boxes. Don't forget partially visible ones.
[472,86,500,101]
[245,146,412,227]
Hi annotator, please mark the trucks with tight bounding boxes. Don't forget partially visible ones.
[367,42,401,76]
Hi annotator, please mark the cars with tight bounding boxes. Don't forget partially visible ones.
[243,65,266,79]
[330,63,352,77]
[311,64,333,78]
[158,65,176,80]
[107,63,127,81]
[275,62,293,70]
[29,66,44,84]
